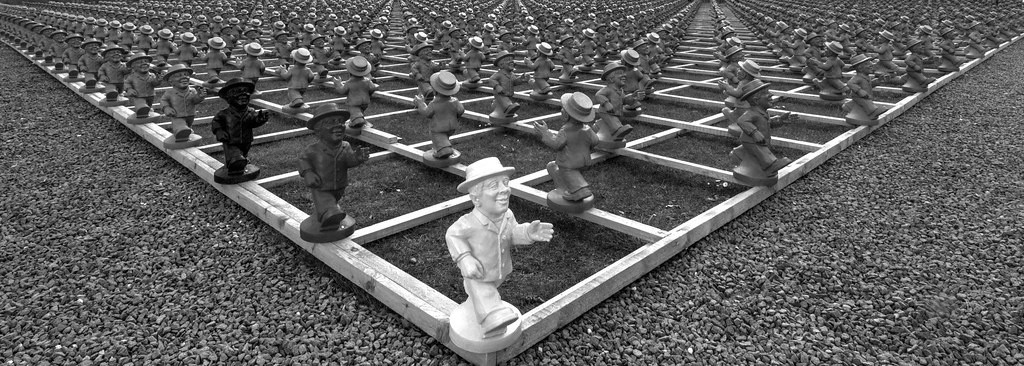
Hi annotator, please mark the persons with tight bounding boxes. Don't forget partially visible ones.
[0,1,1024,231]
[445,157,554,339]
